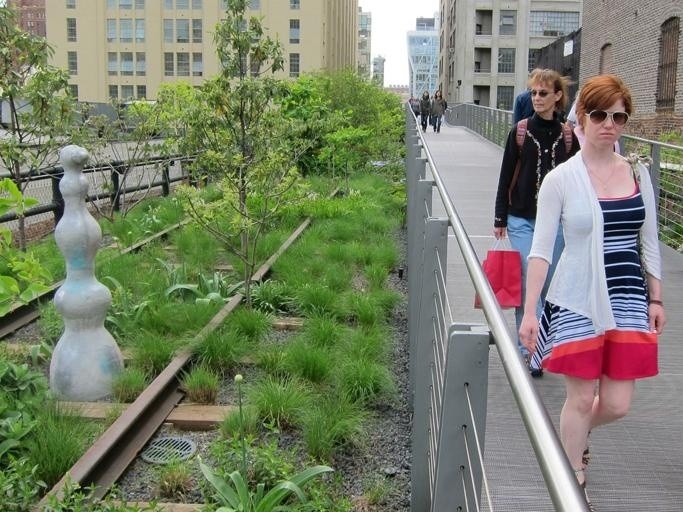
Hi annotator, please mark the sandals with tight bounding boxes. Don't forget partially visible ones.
[573,426,594,512]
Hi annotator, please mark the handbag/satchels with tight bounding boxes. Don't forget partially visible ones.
[475,251,522,310]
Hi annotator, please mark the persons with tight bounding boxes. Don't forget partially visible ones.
[431,90,447,133]
[510,68,542,127]
[407,94,433,117]
[514,74,669,512]
[564,90,583,131]
[418,90,431,133]
[493,70,582,378]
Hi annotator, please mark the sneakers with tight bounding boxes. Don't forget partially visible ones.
[532,369,543,377]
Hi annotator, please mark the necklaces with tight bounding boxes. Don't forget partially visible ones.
[582,153,617,191]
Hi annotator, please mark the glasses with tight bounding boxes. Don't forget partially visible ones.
[585,110,629,127]
[529,90,555,97]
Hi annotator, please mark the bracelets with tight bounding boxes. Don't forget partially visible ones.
[648,299,664,308]
